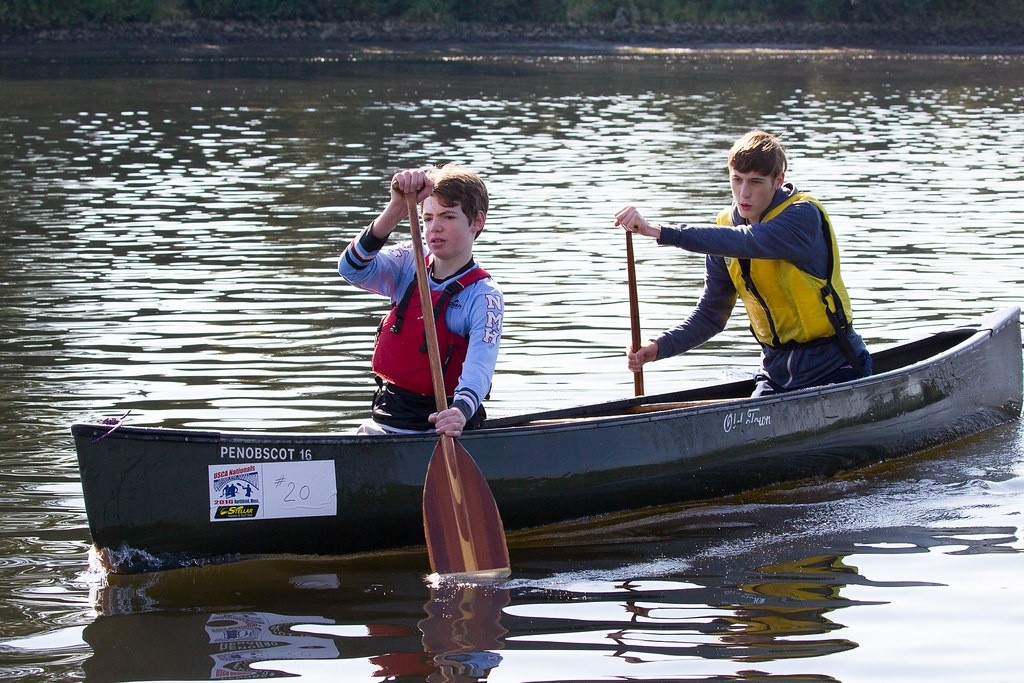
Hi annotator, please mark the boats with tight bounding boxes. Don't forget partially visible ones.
[69,304,1024,582]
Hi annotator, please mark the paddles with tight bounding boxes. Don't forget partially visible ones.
[618,218,646,396]
[390,174,512,582]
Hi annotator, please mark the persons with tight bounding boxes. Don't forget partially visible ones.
[615,129,873,398]
[338,170,504,437]
